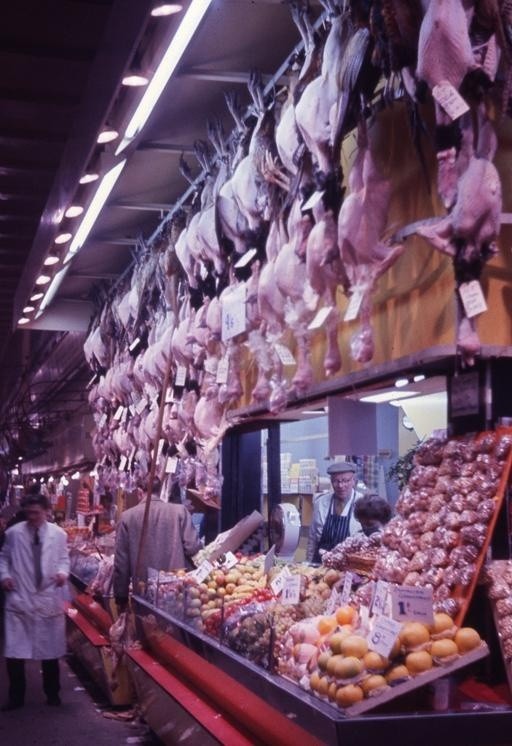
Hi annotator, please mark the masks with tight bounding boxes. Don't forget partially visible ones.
[363,526,381,537]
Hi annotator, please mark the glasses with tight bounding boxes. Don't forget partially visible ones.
[330,475,354,483]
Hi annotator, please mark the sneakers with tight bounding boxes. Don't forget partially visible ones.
[2,693,60,711]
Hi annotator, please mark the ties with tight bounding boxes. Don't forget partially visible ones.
[33,528,40,543]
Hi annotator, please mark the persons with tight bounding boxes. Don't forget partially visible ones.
[353,492,391,537]
[113,474,204,621]
[306,462,367,569]
[1,492,71,713]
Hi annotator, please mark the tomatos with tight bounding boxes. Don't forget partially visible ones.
[204,588,272,637]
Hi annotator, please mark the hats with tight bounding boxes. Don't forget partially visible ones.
[327,463,356,473]
